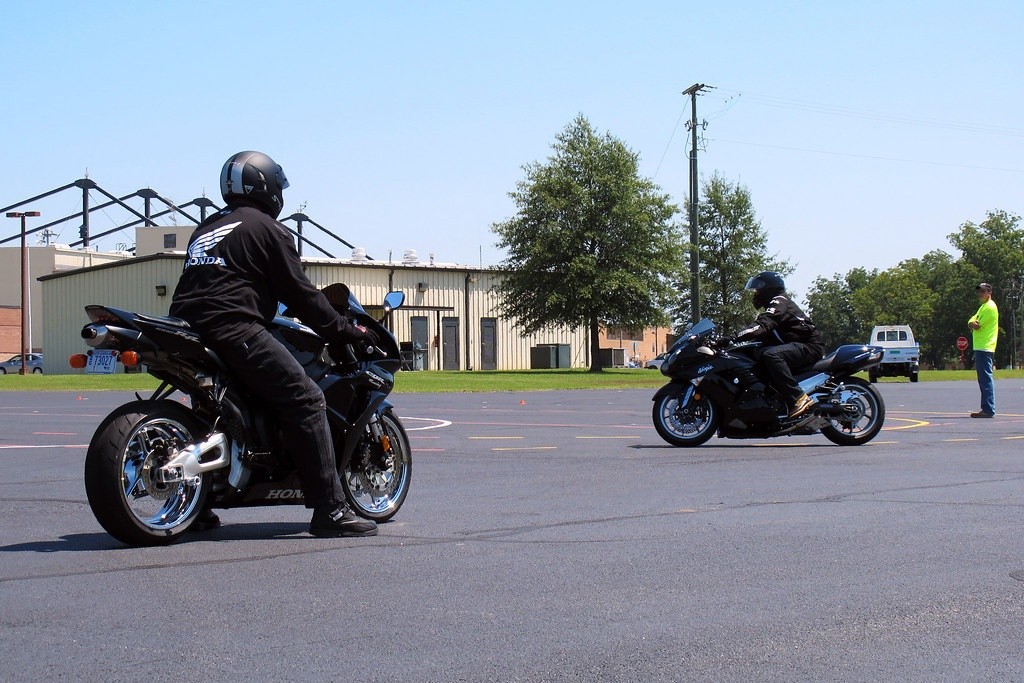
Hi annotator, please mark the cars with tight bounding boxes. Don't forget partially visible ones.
[0,352,43,375]
[645,352,668,370]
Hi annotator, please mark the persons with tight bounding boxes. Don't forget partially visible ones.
[168,151,381,537]
[967,282,999,418]
[717,272,827,417]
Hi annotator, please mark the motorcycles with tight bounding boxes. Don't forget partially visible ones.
[648,317,885,447]
[69,282,412,548]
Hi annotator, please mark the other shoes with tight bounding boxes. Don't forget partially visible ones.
[971,410,993,418]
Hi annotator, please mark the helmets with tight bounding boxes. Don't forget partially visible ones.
[220,151,290,220]
[745,272,785,310]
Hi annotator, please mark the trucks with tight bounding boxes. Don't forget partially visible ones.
[866,324,920,383]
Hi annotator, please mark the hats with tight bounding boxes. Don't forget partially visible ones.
[976,282,992,292]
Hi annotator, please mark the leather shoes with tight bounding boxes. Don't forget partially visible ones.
[788,395,813,418]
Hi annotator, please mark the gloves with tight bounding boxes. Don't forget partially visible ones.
[717,336,734,349]
[350,325,381,346]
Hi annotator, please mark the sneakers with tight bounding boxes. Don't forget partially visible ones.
[310,500,378,537]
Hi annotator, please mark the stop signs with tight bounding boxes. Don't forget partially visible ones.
[957,337,968,351]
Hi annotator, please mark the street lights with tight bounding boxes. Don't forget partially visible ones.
[6,211,41,375]
